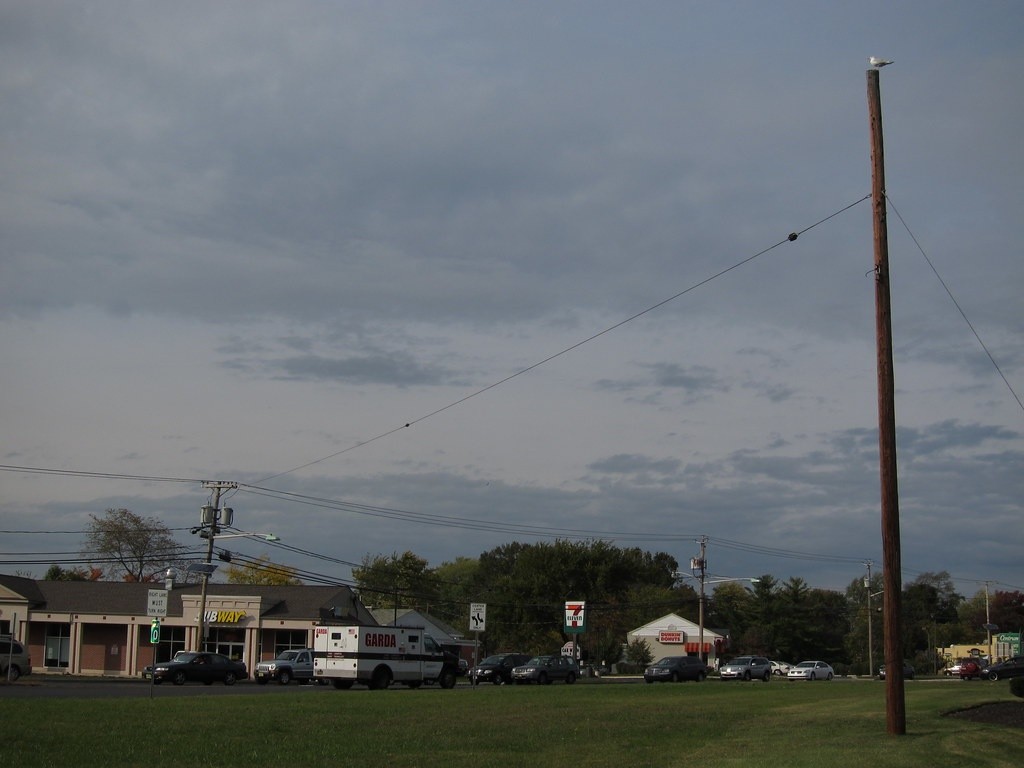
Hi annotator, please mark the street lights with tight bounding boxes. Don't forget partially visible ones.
[196,533,280,651]
[671,571,761,661]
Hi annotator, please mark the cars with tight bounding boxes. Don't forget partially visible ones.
[718,656,772,682]
[787,661,834,681]
[468,653,531,685]
[0,634,32,681]
[980,656,1024,682]
[769,661,791,675]
[644,656,708,683]
[960,658,981,679]
[173,651,204,663]
[142,652,248,685]
[943,658,988,676]
[444,650,468,676]
[511,655,581,684]
[253,648,329,685]
[879,661,915,680]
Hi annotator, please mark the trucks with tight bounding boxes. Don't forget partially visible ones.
[312,624,468,690]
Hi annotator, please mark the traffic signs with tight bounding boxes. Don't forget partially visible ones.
[469,603,486,631]
